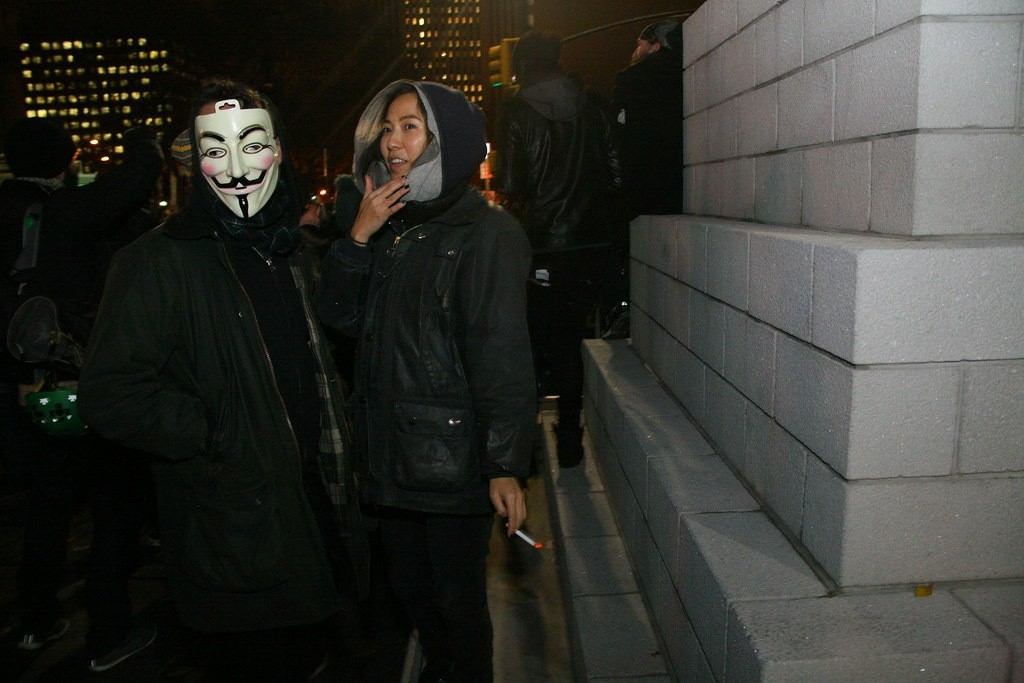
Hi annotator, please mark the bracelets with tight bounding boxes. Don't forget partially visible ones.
[349,237,369,245]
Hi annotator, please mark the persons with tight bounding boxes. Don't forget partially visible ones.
[320,175,362,252]
[0,122,198,672]
[79,81,390,683]
[315,77,537,683]
[494,18,683,477]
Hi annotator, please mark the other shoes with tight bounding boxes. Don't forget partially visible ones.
[555,420,584,468]
[137,519,163,547]
[17,605,84,649]
[600,295,631,341]
[275,647,329,683]
[86,623,157,672]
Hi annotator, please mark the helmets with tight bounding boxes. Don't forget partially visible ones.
[25,390,90,436]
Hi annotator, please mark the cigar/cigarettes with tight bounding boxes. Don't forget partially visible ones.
[506,523,542,548]
